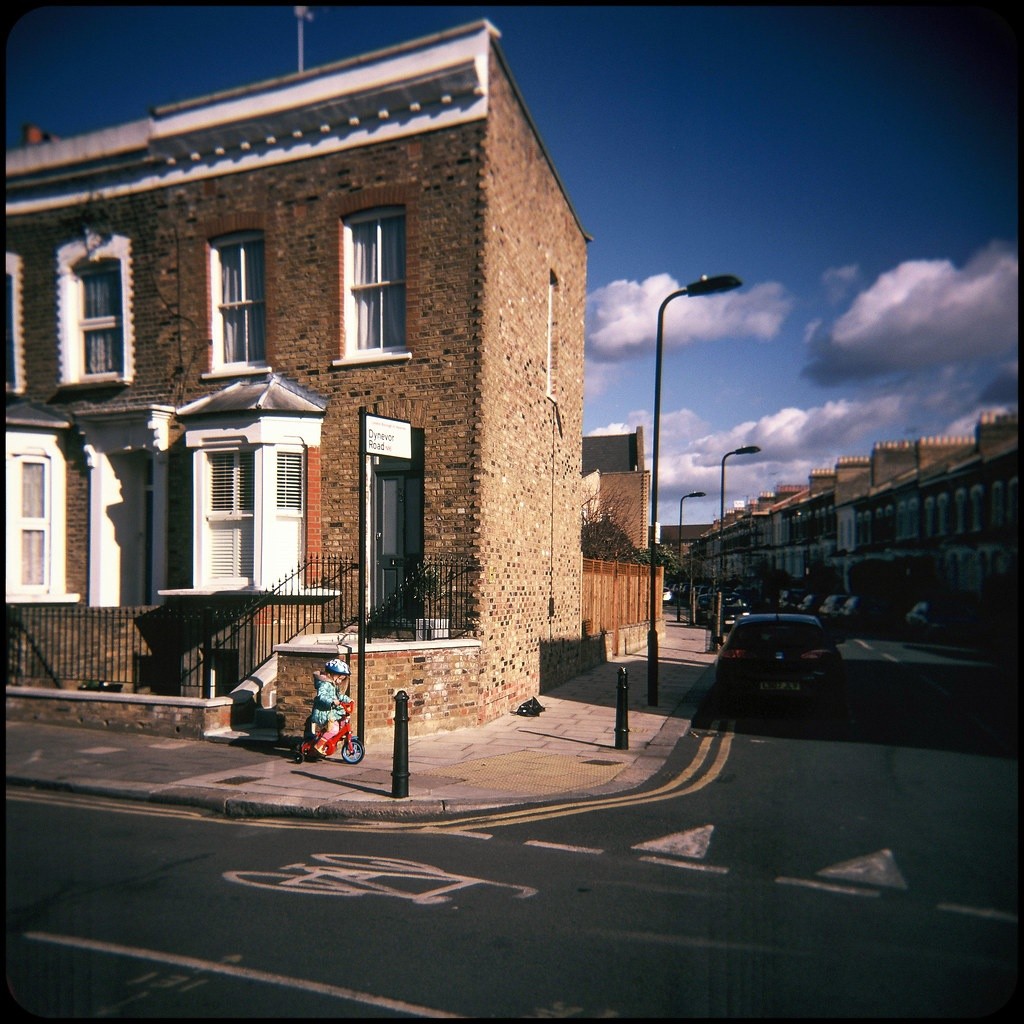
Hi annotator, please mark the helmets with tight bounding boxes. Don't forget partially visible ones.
[325,659,349,674]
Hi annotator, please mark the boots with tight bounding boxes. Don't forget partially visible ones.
[312,738,327,756]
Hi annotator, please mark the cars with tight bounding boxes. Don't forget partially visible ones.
[711,611,847,721]
[663,582,982,642]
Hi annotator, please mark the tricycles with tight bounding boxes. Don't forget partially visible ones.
[289,700,366,764]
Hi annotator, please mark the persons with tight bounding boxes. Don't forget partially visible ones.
[310,660,354,756]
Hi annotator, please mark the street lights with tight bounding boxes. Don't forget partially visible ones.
[718,445,762,642]
[676,492,706,623]
[644,273,744,707]
